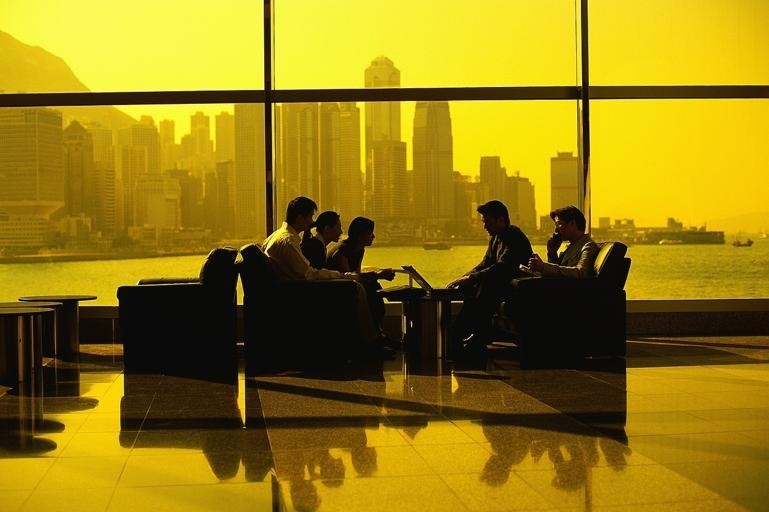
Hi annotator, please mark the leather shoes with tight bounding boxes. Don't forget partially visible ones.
[462,333,491,344]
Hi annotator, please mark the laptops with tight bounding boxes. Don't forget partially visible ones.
[401,265,461,296]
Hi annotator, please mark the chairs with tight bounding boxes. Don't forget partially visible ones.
[512,241,632,371]
[116,242,375,380]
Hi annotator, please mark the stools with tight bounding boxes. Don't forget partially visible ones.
[2,294,97,452]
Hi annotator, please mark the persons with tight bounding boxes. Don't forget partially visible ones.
[300,210,343,270]
[444,200,535,371]
[327,215,397,348]
[528,204,600,281]
[261,196,379,343]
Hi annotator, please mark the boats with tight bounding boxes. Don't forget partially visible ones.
[423,236,453,250]
[732,233,754,247]
